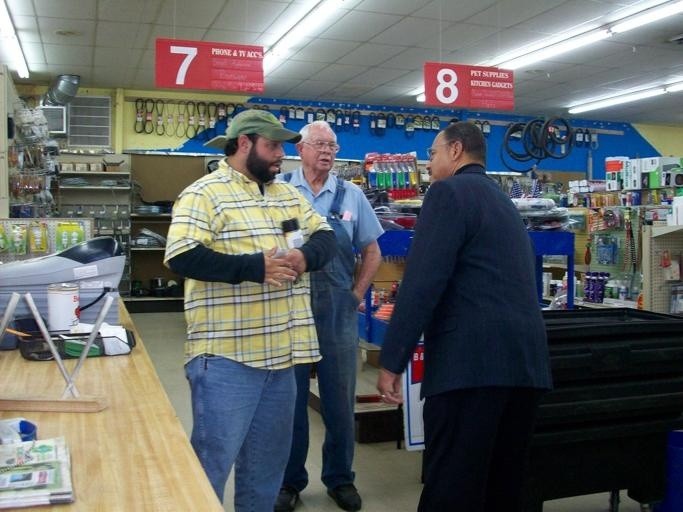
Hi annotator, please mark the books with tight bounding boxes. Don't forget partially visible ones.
[0,436,75,509]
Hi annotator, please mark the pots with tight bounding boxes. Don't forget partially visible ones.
[150,276,167,289]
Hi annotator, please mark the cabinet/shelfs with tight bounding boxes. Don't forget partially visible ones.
[309,227,420,444]
[129,213,184,313]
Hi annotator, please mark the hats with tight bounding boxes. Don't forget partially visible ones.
[226,109,302,144]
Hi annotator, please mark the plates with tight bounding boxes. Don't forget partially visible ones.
[133,204,160,214]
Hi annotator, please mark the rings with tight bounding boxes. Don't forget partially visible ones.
[381,395,386,399]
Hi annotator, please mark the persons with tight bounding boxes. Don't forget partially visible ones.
[271,119,383,512]
[374,121,555,512]
[163,107,339,512]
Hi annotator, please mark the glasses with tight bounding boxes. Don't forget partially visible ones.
[426,138,453,156]
[299,142,340,154]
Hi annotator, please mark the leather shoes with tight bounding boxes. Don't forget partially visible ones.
[327,486,361,512]
[275,490,299,511]
